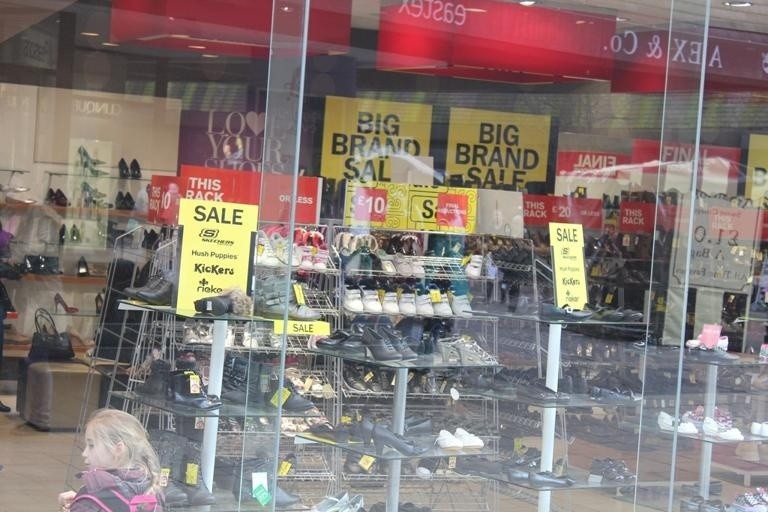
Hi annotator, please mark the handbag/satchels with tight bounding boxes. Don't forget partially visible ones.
[29,332,75,360]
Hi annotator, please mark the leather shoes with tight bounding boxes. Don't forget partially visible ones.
[102,222,132,247]
[116,191,134,209]
[46,188,72,207]
[60,223,80,244]
[95,293,103,314]
[118,158,142,178]
[77,256,89,276]
[0,402,11,411]
[0,183,30,193]
[5,196,37,205]
[0,254,63,280]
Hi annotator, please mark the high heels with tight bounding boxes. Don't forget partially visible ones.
[79,146,107,175]
[54,293,79,313]
[80,181,114,209]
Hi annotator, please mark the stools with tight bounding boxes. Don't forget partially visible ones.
[16,357,103,436]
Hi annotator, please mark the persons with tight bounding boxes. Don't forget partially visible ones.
[0,282,15,411]
[58,409,167,512]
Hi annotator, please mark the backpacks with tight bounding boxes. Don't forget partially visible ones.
[64,483,166,511]
